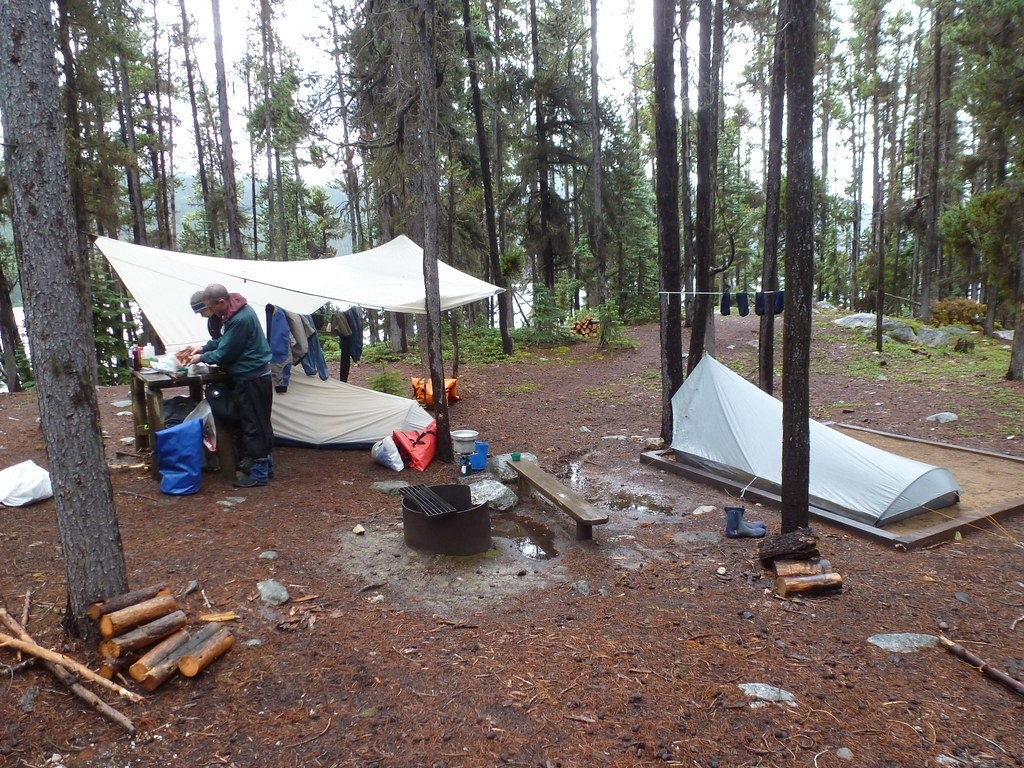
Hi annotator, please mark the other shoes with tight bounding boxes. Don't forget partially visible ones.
[266,470,274,478]
[232,478,267,487]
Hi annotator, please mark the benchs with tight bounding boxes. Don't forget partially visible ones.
[506,460,609,540]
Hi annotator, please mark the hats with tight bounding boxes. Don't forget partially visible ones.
[190,291,208,313]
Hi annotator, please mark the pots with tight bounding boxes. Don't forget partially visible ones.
[451,430,479,453]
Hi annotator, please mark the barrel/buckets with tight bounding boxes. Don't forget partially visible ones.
[460,442,489,470]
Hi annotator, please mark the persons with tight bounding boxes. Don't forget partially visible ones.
[182,283,275,488]
[190,290,246,470]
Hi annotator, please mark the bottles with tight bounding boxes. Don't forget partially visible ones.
[128,346,133,367]
[140,347,149,367]
[132,344,141,371]
[144,342,155,359]
[461,459,472,476]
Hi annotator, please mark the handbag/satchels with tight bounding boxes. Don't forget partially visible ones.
[183,399,216,451]
[411,378,459,408]
[393,420,436,472]
[371,436,404,472]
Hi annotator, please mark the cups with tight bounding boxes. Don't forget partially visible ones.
[208,367,219,373]
[511,452,520,461]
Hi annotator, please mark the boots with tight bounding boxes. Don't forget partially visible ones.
[724,507,766,539]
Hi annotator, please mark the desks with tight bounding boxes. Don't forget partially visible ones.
[130,366,236,474]
